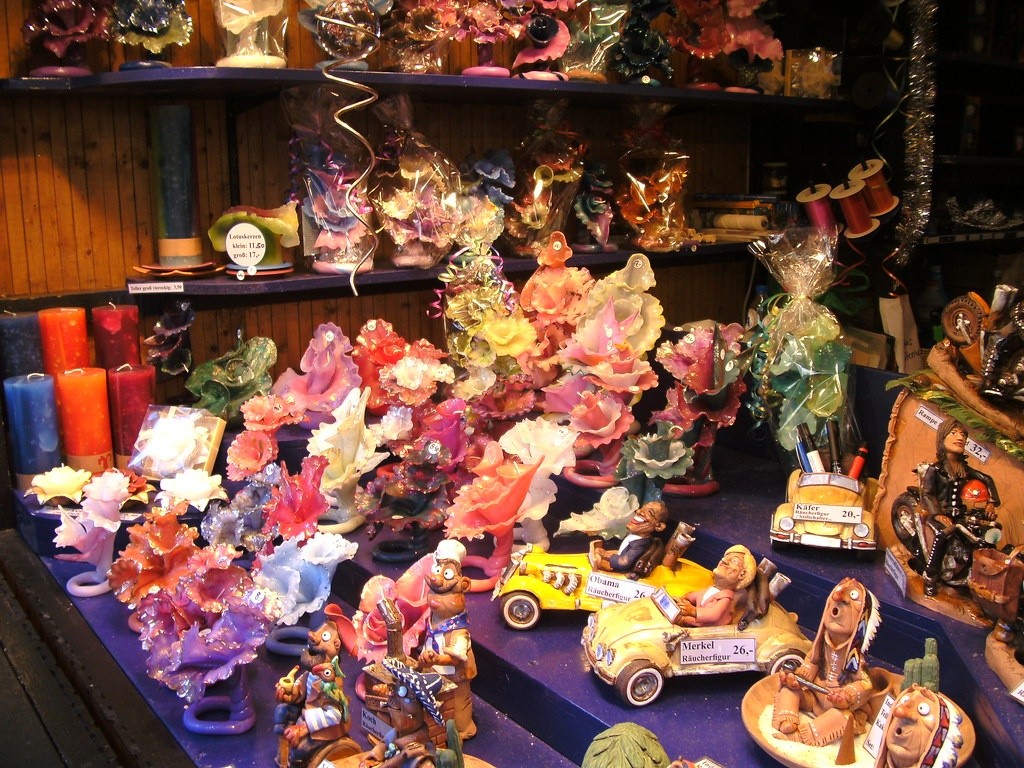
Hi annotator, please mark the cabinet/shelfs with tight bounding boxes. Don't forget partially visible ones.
[0,69,856,326]
[916,10,1024,248]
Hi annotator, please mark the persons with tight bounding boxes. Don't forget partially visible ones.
[875,682,962,768]
[921,416,1001,596]
[416,540,477,741]
[596,499,669,574]
[273,604,359,768]
[772,576,882,748]
[677,544,756,628]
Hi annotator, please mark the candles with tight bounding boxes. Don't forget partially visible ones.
[107,364,155,456]
[92,302,141,368]
[37,307,91,400]
[57,365,114,455]
[4,373,59,475]
[0,311,44,380]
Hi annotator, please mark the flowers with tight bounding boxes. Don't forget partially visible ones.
[23,0,852,734]
